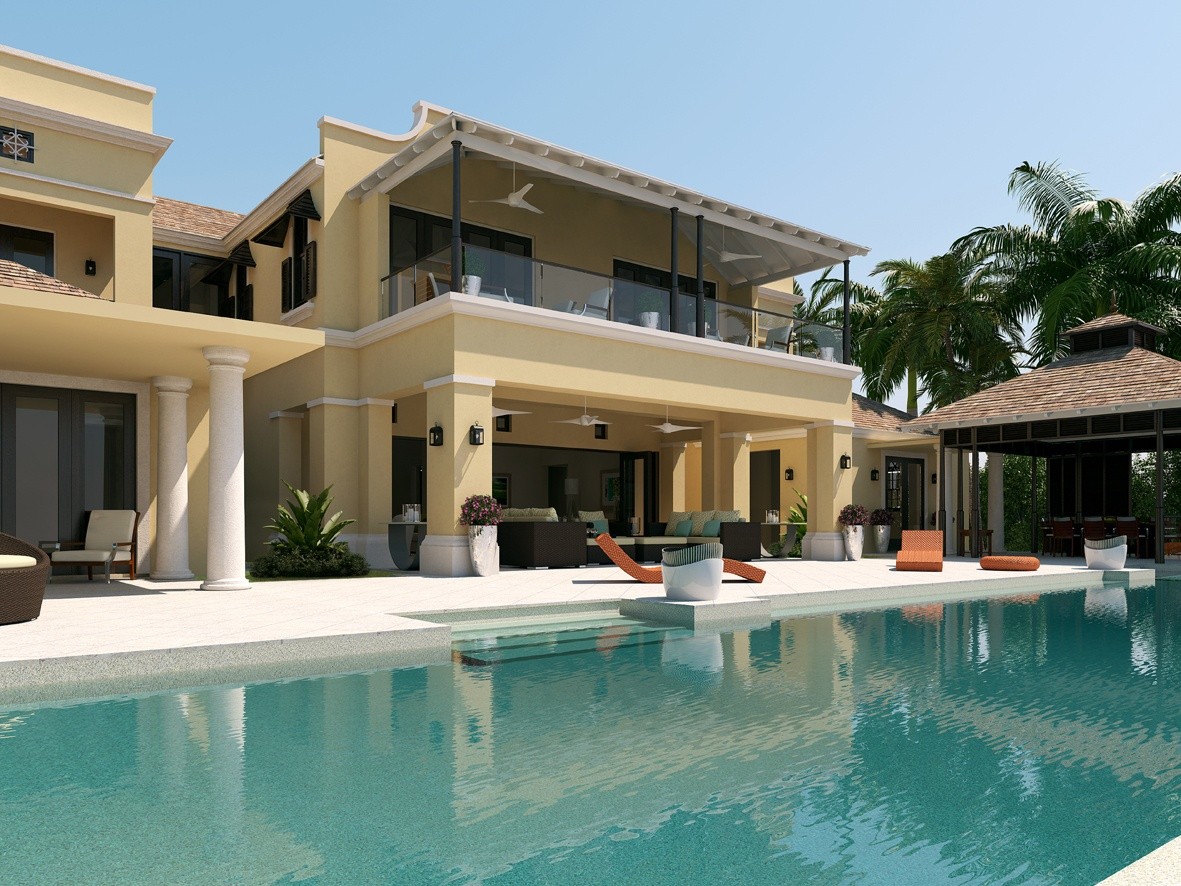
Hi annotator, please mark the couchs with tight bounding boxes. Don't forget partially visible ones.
[497,521,761,569]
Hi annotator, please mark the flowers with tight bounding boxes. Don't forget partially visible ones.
[458,494,894,534]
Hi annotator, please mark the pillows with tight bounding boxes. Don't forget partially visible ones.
[504,508,743,537]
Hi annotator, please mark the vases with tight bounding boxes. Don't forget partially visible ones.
[466,525,891,576]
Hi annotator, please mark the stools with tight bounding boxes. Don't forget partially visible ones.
[0,532,51,625]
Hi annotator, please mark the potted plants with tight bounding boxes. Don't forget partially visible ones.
[441,249,838,360]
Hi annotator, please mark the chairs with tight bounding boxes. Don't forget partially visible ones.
[407,273,794,360]
[49,509,140,584]
[1041,516,1140,562]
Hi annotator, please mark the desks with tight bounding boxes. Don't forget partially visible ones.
[388,522,427,570]
[1040,526,1156,558]
[586,538,635,565]
[962,530,994,558]
[760,522,808,558]
[635,538,688,563]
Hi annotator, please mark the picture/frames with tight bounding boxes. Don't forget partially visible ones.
[600,470,621,507]
[492,472,512,508]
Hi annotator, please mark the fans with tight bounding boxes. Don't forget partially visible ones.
[467,183,762,265]
[491,396,704,434]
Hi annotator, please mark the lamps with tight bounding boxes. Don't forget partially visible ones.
[428,424,941,483]
